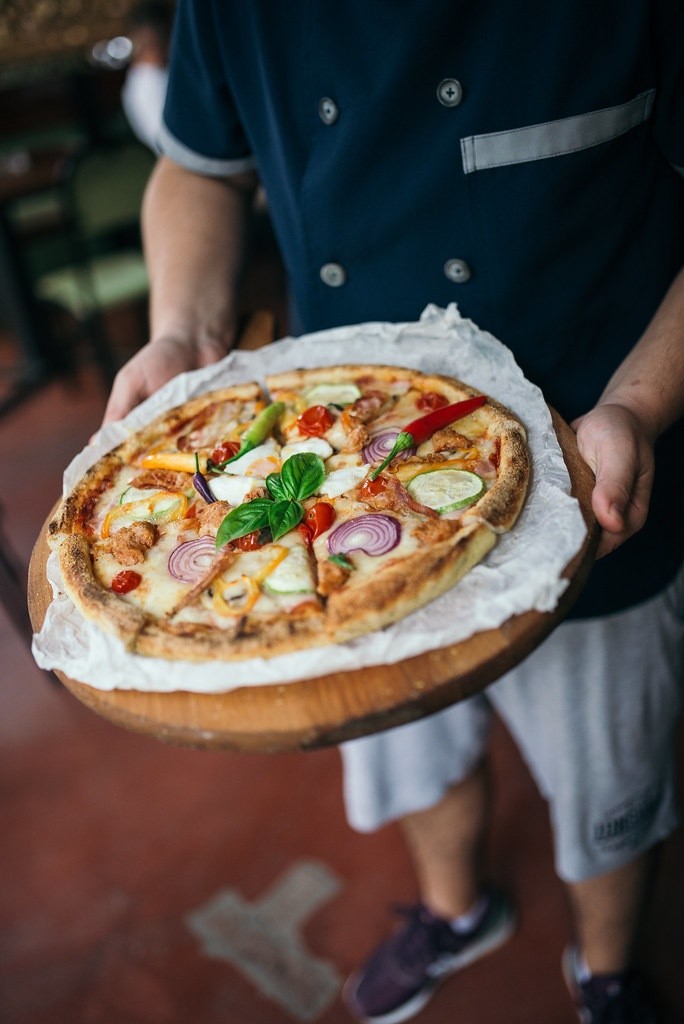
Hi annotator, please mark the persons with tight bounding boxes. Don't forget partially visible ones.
[101,0,684,1024]
[122,0,175,158]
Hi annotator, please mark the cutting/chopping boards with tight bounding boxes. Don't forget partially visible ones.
[26,384,601,750]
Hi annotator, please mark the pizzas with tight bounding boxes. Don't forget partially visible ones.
[55,364,530,657]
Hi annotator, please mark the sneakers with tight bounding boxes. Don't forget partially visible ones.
[562,945,655,1024]
[340,882,515,1023]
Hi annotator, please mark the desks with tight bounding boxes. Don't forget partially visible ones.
[0,38,131,390]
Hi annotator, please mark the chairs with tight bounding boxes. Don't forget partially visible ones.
[30,137,156,391]
